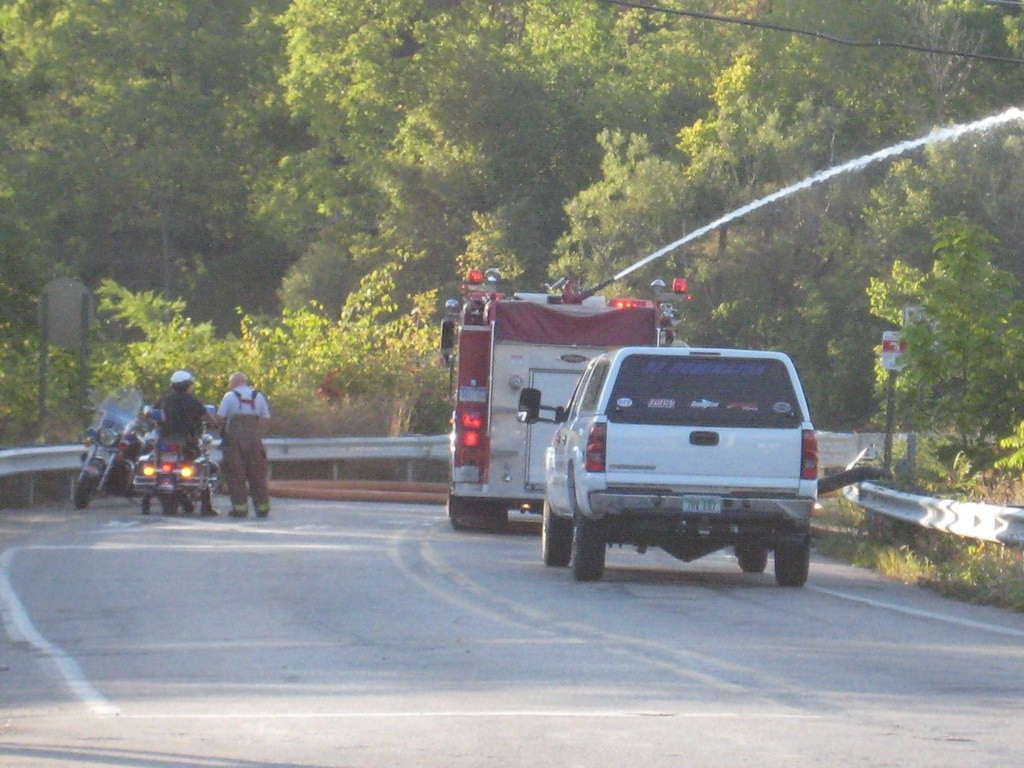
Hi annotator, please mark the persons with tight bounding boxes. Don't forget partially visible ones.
[141,371,221,516]
[217,372,270,518]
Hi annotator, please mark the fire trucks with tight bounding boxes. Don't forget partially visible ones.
[439,269,696,532]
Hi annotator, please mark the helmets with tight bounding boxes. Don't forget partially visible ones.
[169,370,195,388]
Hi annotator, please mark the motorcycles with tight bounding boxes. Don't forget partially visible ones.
[70,386,225,518]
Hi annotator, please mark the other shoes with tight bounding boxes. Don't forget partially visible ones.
[201,506,219,516]
[256,511,268,517]
[229,510,247,516]
[142,498,151,515]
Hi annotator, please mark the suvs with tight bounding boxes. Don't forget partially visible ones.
[516,345,819,581]
